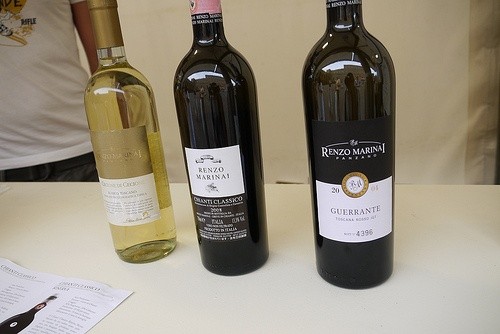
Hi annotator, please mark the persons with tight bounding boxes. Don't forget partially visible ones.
[0,0,100,182]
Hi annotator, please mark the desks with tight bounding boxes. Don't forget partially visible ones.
[0,182,500,334]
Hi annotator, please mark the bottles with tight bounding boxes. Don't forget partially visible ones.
[84,0,177,264]
[302,2,396,290]
[173,0,270,276]
